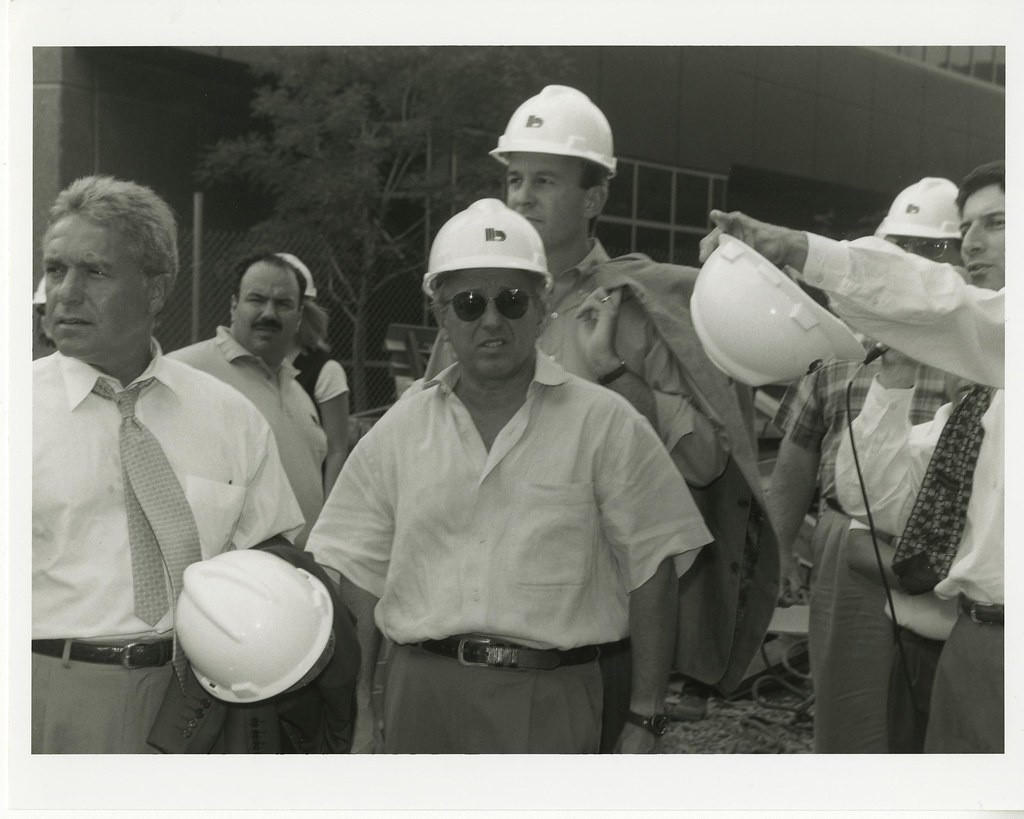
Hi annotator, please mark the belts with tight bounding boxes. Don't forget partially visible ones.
[956,591,1004,627]
[412,631,600,669]
[825,498,845,515]
[32,634,174,669]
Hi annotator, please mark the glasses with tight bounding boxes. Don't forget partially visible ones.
[443,288,539,322]
[897,236,958,258]
[36,304,45,316]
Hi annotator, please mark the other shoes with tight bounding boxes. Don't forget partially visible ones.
[670,684,709,719]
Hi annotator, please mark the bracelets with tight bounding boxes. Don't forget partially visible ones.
[597,361,626,385]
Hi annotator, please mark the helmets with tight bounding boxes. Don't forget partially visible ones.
[273,253,317,297]
[34,275,47,304]
[690,233,867,387]
[883,177,965,239]
[173,549,334,703]
[488,84,616,180]
[422,198,554,300]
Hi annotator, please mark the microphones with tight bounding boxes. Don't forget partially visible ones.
[861,267,973,365]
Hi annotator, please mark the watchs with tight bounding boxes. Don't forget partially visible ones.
[626,710,669,737]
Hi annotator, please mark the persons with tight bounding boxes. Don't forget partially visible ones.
[422,84,728,583]
[764,176,961,753]
[164,252,349,754]
[304,197,715,754]
[700,159,1006,754]
[32,175,307,754]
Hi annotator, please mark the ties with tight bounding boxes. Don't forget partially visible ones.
[91,376,202,696]
[890,381,994,594]
